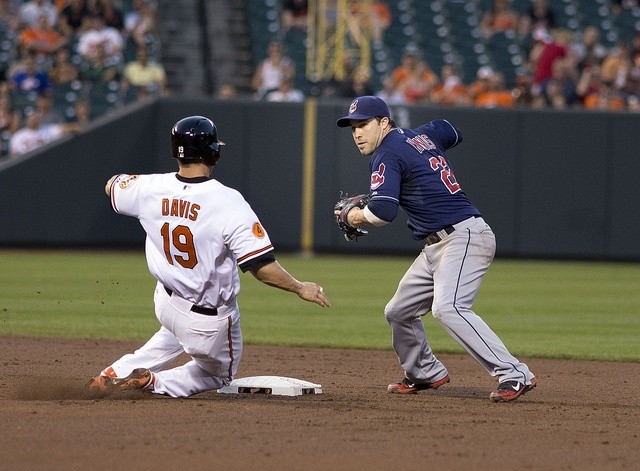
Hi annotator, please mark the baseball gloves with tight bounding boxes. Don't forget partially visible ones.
[334,194,370,241]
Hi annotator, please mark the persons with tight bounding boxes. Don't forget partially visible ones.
[252,0,311,100]
[212,82,237,100]
[1,2,167,155]
[348,0,640,111]
[335,96,537,402]
[87,116,331,394]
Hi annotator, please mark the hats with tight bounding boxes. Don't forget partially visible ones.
[336,95,391,127]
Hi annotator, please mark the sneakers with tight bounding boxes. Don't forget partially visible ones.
[113,366,155,393]
[489,372,537,403]
[387,372,451,395]
[80,368,117,395]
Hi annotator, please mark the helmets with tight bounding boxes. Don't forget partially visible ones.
[170,113,227,161]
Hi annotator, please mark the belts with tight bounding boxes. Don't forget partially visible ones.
[162,284,220,317]
[424,224,456,245]
